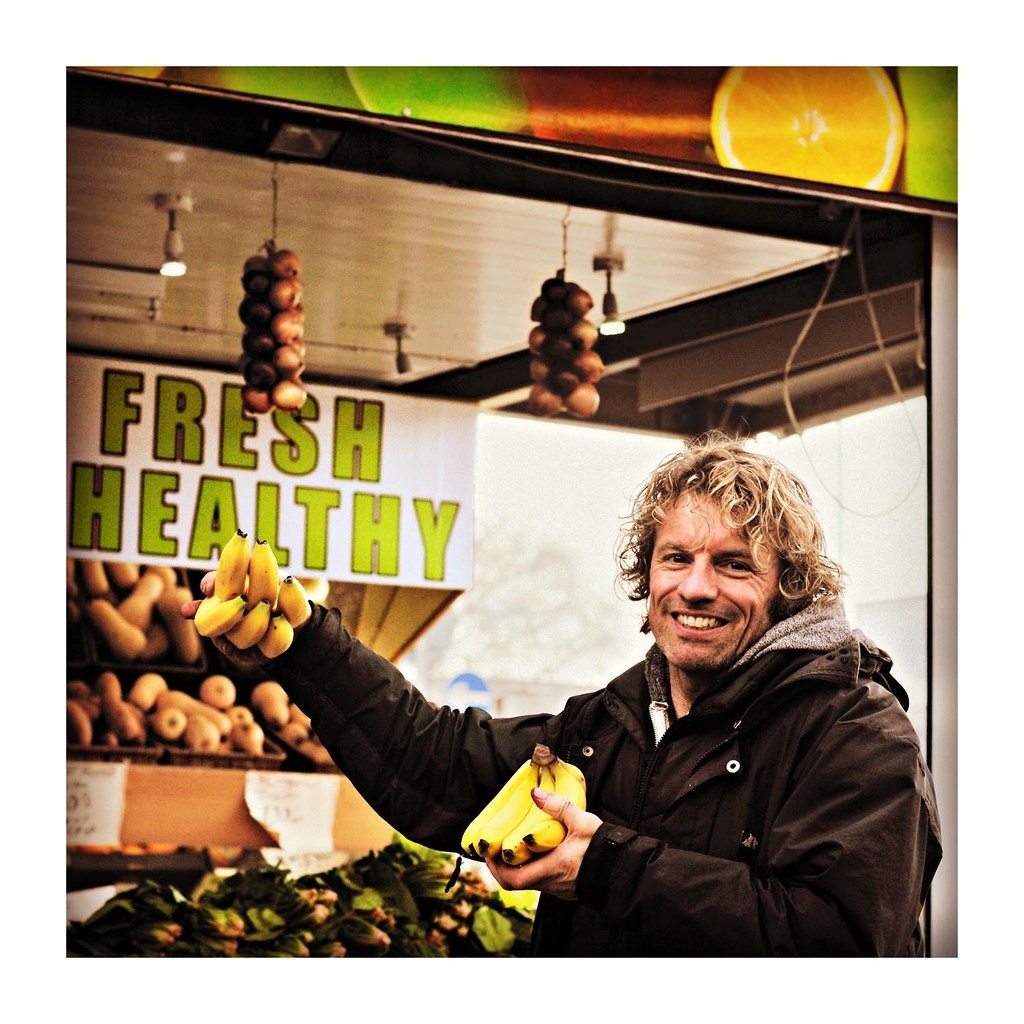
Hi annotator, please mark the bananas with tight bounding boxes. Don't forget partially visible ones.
[460,744,586,862]
[191,530,311,657]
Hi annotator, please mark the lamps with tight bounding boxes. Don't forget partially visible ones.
[385,324,412,374]
[67,264,166,324]
[594,256,626,335]
[154,193,192,276]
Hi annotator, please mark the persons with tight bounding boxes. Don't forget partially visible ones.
[179,443,944,958]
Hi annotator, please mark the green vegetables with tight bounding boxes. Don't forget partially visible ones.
[66,842,534,959]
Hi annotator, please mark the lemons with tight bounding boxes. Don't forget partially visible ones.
[707,65,905,192]
